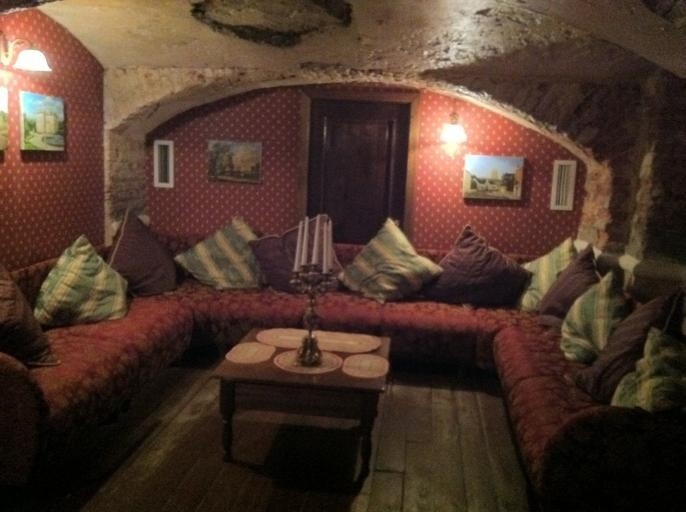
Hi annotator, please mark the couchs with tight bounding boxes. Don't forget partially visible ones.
[151,236,540,375]
[0,242,193,512]
[488,252,686,512]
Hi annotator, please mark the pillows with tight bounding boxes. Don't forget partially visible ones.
[525,236,686,410]
[0,210,176,368]
[422,223,533,307]
[339,219,442,307]
[177,214,264,289]
[245,225,344,293]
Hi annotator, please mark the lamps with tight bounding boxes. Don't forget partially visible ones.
[0,33,53,73]
[441,98,468,156]
